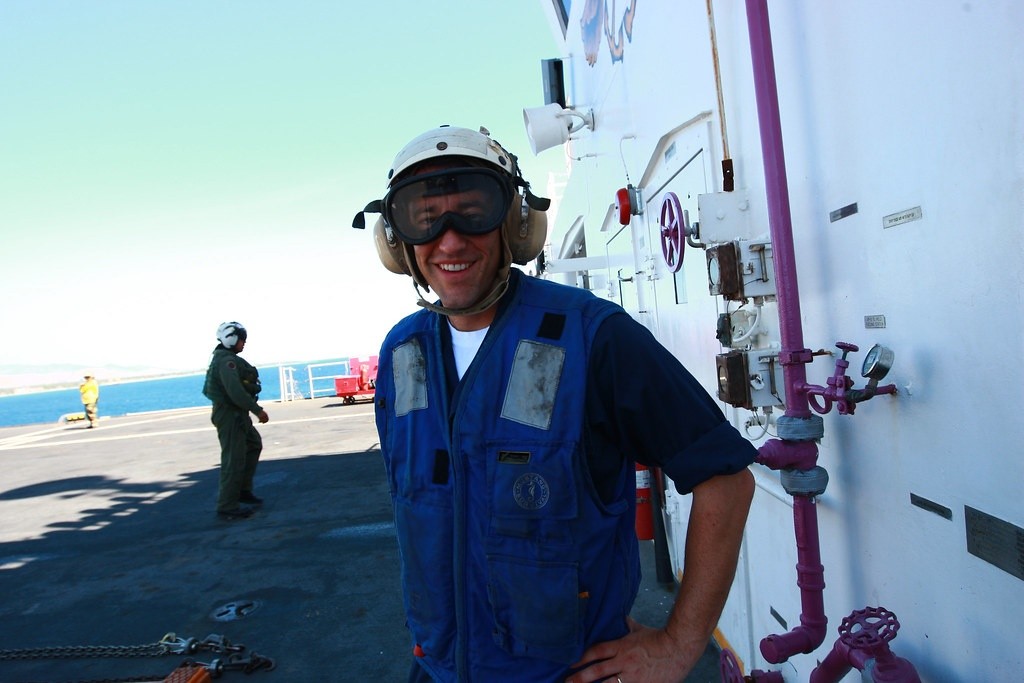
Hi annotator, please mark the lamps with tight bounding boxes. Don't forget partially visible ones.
[522,102,595,157]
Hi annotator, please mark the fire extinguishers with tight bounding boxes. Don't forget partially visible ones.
[634,462,663,539]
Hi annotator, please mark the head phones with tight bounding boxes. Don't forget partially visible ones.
[372,187,548,275]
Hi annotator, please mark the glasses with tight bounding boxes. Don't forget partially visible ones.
[381,167,513,245]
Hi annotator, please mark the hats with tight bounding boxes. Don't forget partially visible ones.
[83,372,92,378]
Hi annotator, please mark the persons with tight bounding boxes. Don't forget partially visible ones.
[352,124,758,683]
[202,321,271,520]
[79,370,100,428]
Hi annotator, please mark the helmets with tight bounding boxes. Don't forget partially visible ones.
[217,321,247,348]
[382,124,519,245]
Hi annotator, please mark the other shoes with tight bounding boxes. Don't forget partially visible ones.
[239,491,264,503]
[217,507,252,520]
